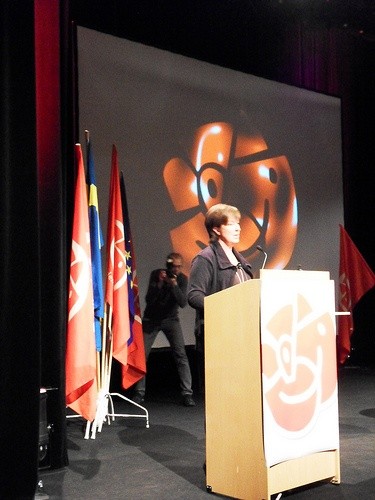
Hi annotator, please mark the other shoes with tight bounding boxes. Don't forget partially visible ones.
[132,393,145,405]
[184,395,196,407]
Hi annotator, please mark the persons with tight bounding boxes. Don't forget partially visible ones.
[131,253,196,406]
[187,203,254,475]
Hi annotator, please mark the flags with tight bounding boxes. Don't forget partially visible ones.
[338,224,375,364]
[65,134,147,424]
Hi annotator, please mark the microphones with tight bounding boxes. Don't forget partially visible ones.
[256,245,268,269]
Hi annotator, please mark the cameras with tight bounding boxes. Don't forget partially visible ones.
[165,259,175,278]
